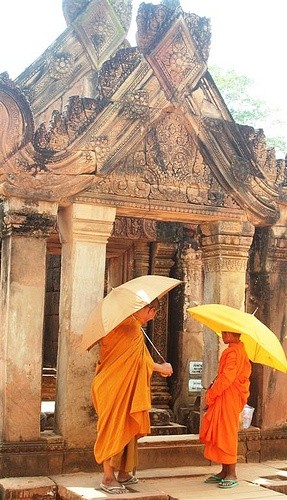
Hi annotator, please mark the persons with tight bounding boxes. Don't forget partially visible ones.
[91,297,173,494]
[199,332,251,489]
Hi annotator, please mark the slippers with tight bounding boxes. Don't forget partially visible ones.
[218,479,239,488]
[204,475,221,482]
[118,476,139,484]
[100,480,130,493]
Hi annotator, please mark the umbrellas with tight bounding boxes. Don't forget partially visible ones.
[186,304,287,389]
[80,274,182,377]
